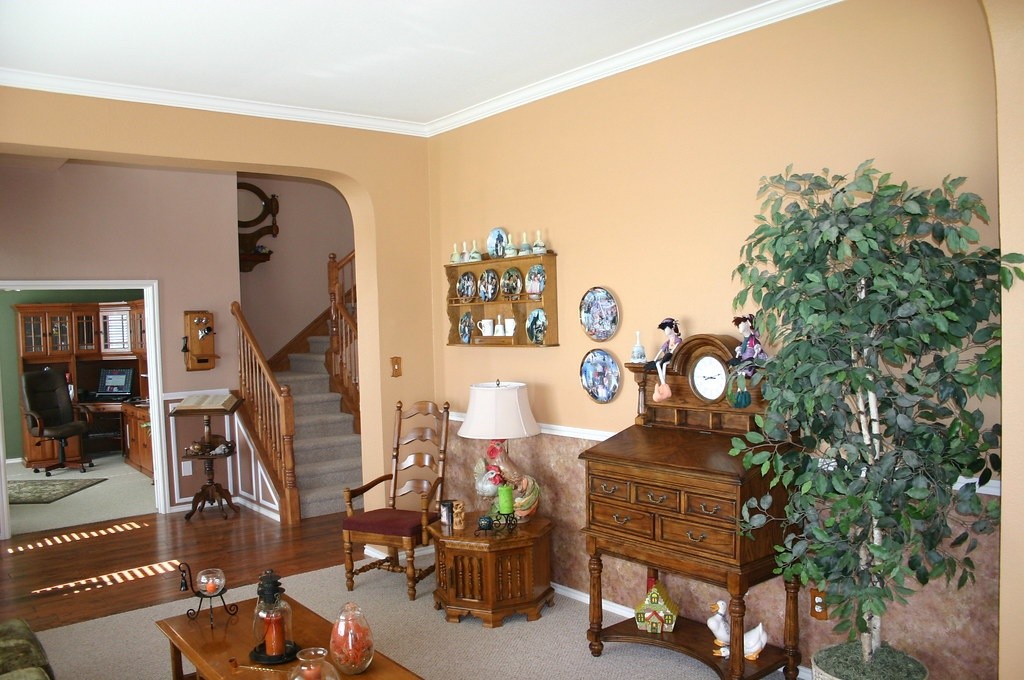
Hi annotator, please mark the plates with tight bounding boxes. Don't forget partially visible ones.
[525,263,546,300]
[579,348,622,404]
[477,269,500,302]
[486,226,508,259]
[456,271,476,303]
[458,311,472,344]
[500,267,523,301]
[578,286,619,342]
[524,308,546,345]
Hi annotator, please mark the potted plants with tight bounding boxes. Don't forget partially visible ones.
[726,158,1023,680]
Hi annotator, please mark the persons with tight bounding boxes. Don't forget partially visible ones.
[479,271,496,300]
[644,318,682,402]
[501,272,520,300]
[527,270,545,299]
[497,230,503,256]
[726,313,767,408]
[459,318,474,343]
[457,276,473,300]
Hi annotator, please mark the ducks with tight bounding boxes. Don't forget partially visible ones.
[712,622,769,660]
[706,600,730,647]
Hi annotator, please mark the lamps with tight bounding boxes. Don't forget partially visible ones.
[457,379,541,454]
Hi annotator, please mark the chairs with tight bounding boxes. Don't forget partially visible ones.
[21,367,94,477]
[343,398,450,600]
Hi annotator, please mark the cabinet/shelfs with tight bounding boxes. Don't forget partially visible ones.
[168,393,245,522]
[10,299,153,481]
[443,249,559,348]
[581,333,802,680]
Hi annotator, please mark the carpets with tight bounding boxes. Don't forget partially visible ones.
[34,547,823,680]
[5,451,157,536]
[7,478,108,504]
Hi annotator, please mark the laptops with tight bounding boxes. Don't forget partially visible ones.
[88,366,134,403]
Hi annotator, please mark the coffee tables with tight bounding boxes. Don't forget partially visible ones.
[154,594,423,680]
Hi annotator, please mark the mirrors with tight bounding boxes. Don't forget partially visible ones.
[237,182,280,272]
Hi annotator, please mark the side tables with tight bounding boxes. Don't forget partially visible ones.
[426,510,556,629]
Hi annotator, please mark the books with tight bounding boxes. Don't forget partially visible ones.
[174,393,238,411]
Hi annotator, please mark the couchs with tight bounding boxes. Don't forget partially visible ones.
[0,617,55,680]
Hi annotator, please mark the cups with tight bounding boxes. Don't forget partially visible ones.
[476,318,494,336]
[505,319,516,336]
[493,325,505,336]
[196,568,226,596]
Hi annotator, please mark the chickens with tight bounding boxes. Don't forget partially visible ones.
[473,457,504,500]
[487,439,540,519]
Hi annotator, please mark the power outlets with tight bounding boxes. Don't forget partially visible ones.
[808,588,829,620]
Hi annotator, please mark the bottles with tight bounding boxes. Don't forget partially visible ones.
[460,241,470,263]
[504,233,517,258]
[469,240,482,262]
[449,242,461,264]
[532,230,547,255]
[329,601,374,675]
[517,232,533,256]
[289,647,342,680]
